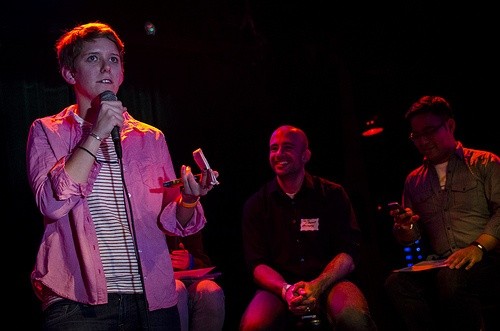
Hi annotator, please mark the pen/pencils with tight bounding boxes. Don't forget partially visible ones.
[163,176,199,187]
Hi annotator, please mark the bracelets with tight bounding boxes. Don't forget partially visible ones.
[181,197,200,208]
[75,146,97,160]
[89,133,103,142]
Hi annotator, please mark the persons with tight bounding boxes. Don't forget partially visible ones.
[383,94,500,331]
[26,22,225,331]
[238,124,377,331]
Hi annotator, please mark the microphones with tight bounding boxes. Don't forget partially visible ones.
[100,90,123,159]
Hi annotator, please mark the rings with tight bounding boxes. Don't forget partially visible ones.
[201,185,206,189]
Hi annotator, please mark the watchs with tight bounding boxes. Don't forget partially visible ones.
[471,242,487,254]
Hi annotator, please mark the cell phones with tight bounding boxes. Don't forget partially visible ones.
[388,202,415,227]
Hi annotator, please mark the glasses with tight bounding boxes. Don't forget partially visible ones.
[408,118,447,141]
